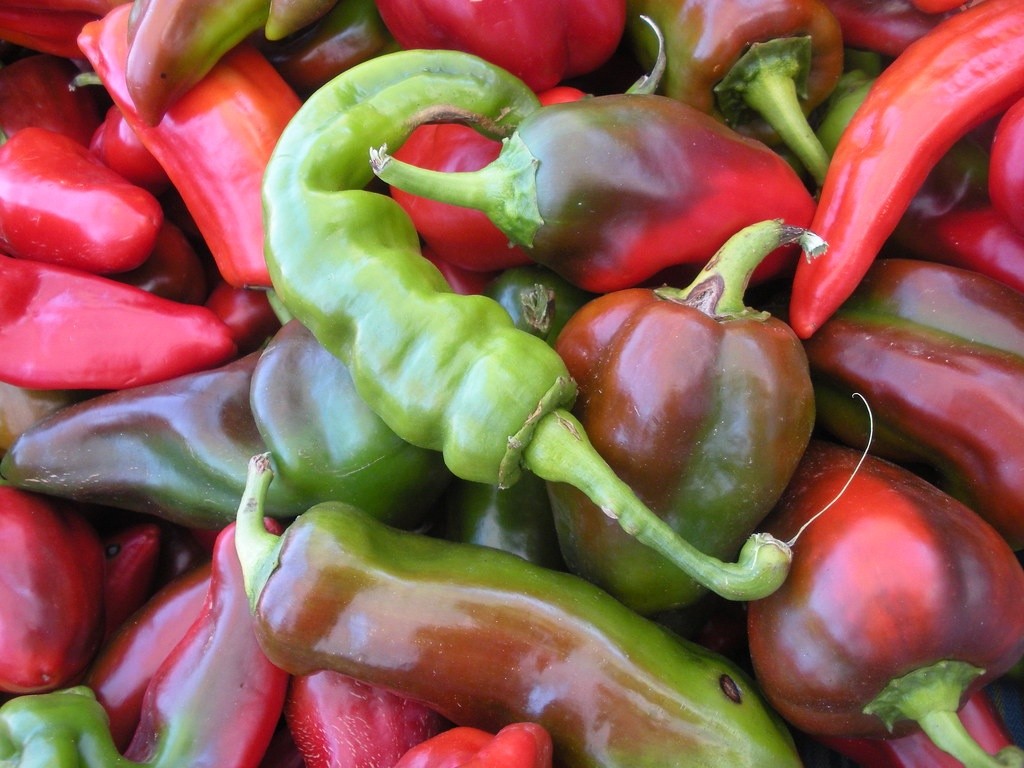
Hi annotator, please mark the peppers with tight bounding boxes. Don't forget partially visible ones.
[0,0,1024,768]
